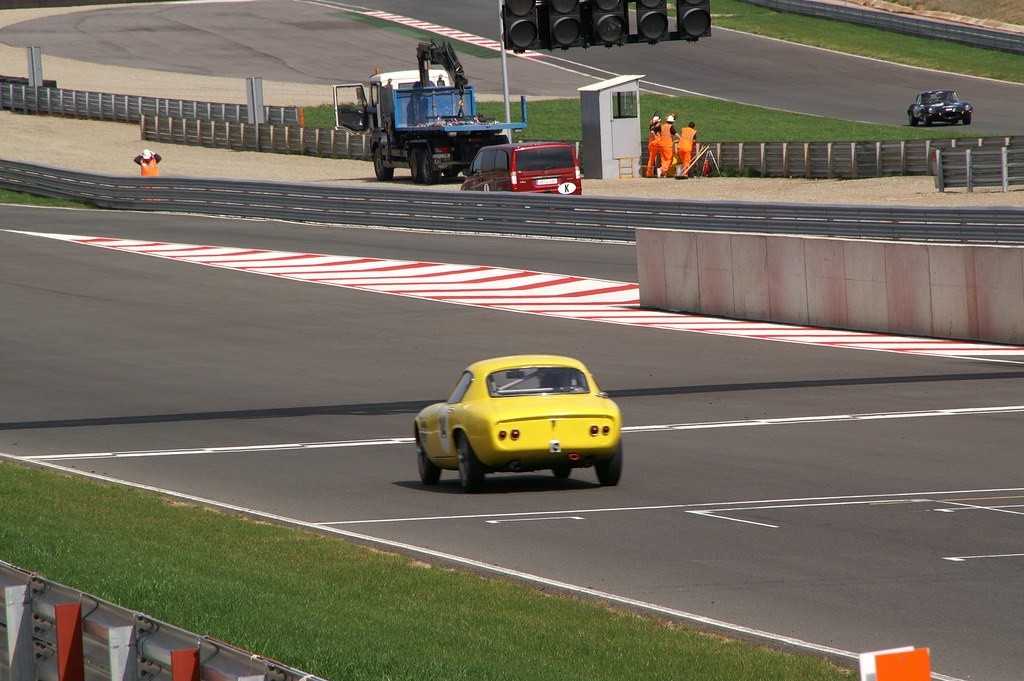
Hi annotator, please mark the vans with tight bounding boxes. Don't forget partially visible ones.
[453,142,582,214]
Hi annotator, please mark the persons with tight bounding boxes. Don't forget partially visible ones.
[677,121,698,177]
[657,115,680,178]
[646,115,662,178]
[135,149,162,202]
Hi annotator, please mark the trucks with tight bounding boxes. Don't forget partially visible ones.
[332,39,528,184]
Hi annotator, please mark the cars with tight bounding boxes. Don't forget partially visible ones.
[907,89,973,126]
[413,354,622,490]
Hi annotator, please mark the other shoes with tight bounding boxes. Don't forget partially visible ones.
[646,176,652,178]
[689,169,694,178]
[657,168,662,178]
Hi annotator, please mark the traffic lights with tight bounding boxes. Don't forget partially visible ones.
[502,0,712,55]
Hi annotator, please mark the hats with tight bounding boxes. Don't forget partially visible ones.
[143,149,151,159]
[650,116,661,123]
[667,115,674,122]
